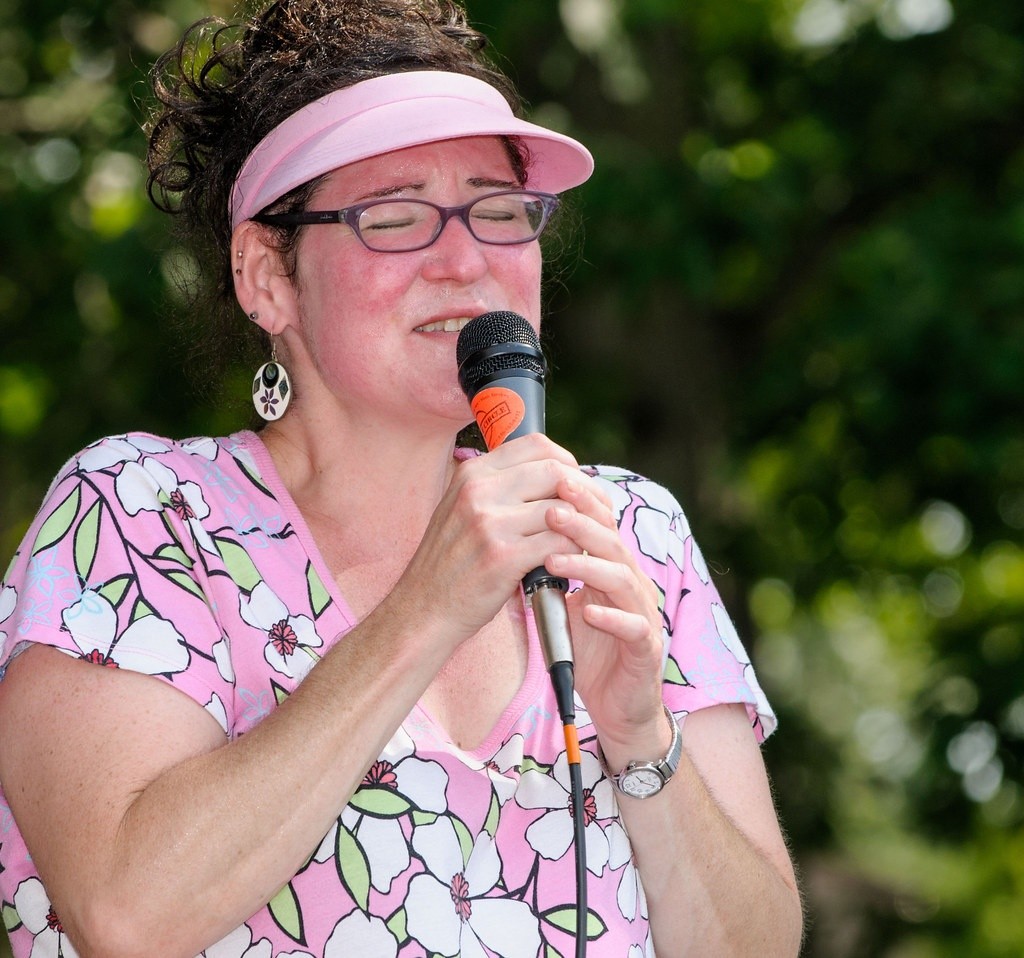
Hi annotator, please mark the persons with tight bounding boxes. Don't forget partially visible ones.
[0,0,802,958]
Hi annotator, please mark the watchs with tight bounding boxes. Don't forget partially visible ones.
[597,704,682,800]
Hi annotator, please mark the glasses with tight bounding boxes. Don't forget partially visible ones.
[248,190,564,252]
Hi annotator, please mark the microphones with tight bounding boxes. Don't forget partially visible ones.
[456,310,580,727]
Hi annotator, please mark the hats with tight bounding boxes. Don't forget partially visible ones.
[226,70,598,231]
